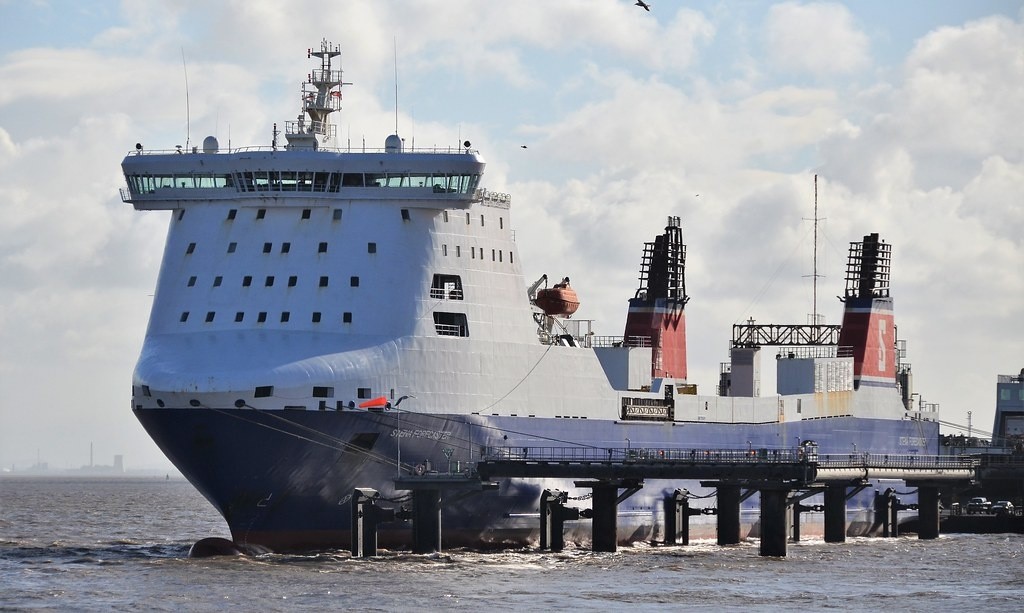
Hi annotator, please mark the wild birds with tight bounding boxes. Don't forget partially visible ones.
[634,0,651,11]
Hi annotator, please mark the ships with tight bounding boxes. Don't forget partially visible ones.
[119,37,1024,547]
